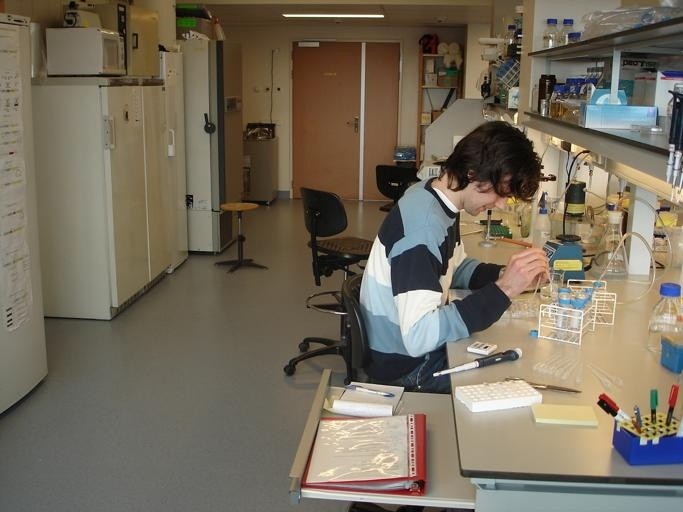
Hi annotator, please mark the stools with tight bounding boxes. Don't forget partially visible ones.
[214,202,268,275]
[283,291,351,385]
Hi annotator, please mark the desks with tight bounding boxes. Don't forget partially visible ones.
[288,188,680,512]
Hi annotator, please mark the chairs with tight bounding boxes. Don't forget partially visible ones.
[339,272,368,385]
[298,165,421,287]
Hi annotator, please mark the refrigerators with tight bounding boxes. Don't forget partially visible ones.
[178,40,246,255]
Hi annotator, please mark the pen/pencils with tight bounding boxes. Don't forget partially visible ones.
[505,377,583,394]
[597,384,679,434]
[344,385,396,397]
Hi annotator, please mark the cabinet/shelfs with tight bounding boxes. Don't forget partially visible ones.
[415,25,468,172]
[518,16,682,187]
[29,78,170,321]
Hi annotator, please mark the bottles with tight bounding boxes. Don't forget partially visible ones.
[555,287,599,333]
[588,211,629,279]
[647,282,683,353]
[543,17,582,49]
[481,75,491,97]
[536,74,556,114]
[504,24,516,57]
[520,201,552,251]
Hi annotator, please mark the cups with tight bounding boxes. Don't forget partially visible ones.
[538,268,565,301]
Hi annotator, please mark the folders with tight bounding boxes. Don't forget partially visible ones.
[301,413,428,497]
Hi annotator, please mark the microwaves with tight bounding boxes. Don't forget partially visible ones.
[44,25,127,78]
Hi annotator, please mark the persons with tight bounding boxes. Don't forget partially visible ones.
[356,119,551,394]
